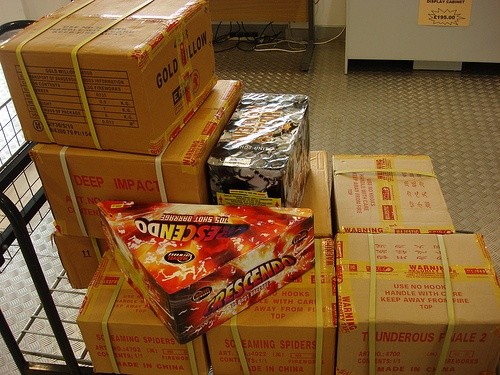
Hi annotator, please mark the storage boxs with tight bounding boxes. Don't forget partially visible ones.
[0,0,500,375]
